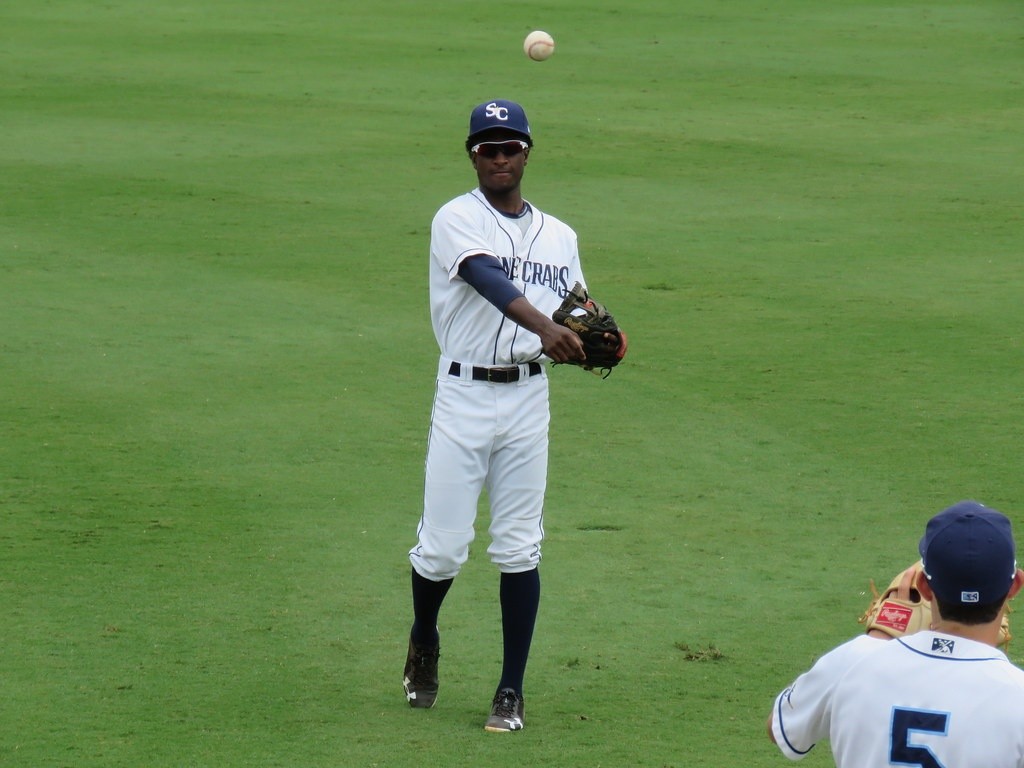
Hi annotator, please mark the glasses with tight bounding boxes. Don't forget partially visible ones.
[471,140,529,158]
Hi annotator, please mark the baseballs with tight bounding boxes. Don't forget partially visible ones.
[523,30,556,63]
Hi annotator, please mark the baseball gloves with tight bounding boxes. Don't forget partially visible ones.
[539,280,628,380]
[856,555,1014,653]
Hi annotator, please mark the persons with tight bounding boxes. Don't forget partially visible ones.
[401,99,627,732]
[767,501,1024,768]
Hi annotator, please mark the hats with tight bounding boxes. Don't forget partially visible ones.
[919,502,1016,607]
[470,98,531,139]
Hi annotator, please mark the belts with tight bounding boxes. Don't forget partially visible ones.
[448,361,542,383]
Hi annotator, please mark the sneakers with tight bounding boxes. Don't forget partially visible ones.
[484,687,525,732]
[403,622,440,709]
